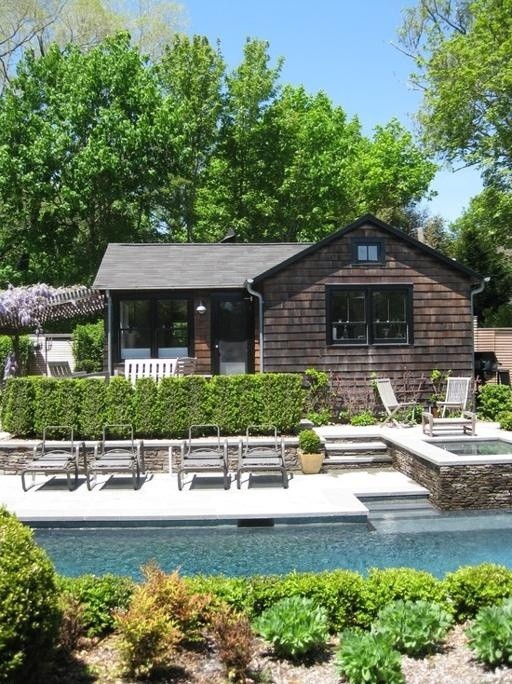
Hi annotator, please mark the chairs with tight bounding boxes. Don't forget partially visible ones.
[85,424,144,490]
[437,377,472,418]
[237,425,288,490]
[376,378,418,429]
[176,424,229,491]
[21,426,84,491]
[48,358,197,387]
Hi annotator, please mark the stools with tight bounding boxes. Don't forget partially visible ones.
[422,412,475,436]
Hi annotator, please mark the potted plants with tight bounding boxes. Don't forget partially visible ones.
[297,429,323,473]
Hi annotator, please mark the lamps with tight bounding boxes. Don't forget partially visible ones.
[196,297,207,315]
